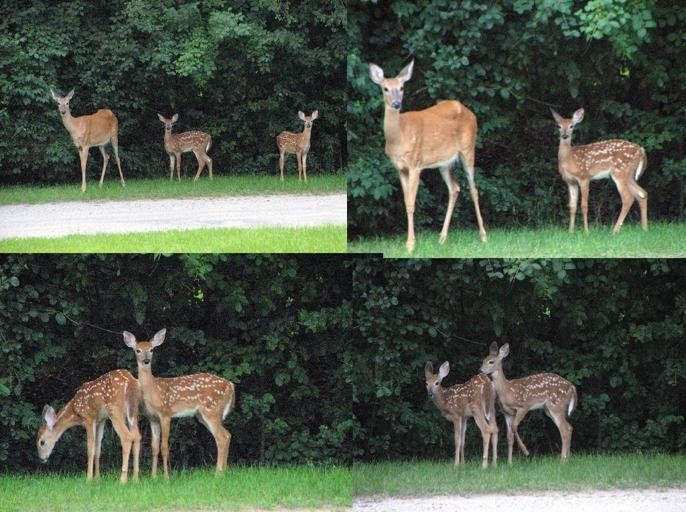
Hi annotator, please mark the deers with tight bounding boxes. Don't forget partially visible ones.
[367,56,488,253]
[120,326,236,480]
[34,367,143,484]
[156,112,214,182]
[547,104,650,236]
[477,339,579,465]
[274,108,319,183]
[422,360,500,470]
[48,87,125,191]
